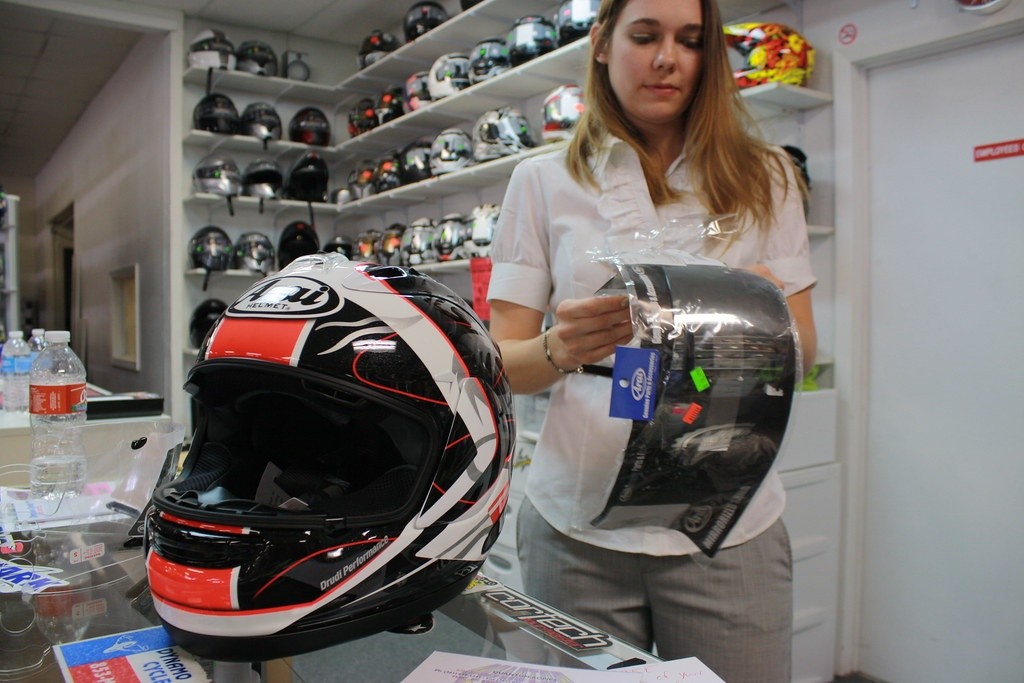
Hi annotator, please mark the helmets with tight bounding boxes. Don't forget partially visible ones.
[373,87,404,127]
[357,29,403,70]
[285,151,329,203]
[426,52,470,100]
[242,102,282,141]
[557,0,602,48]
[189,298,228,349]
[473,104,537,162]
[352,204,502,266]
[346,127,473,199]
[238,41,278,75]
[193,94,241,134]
[348,98,377,138]
[508,13,557,68]
[279,220,320,270]
[540,83,586,144]
[243,160,283,199]
[403,1,449,43]
[289,107,330,147]
[467,39,507,85]
[187,29,238,70]
[193,154,242,196]
[722,21,819,88]
[144,252,517,662]
[235,232,278,273]
[323,235,354,260]
[404,71,433,111]
[188,226,235,270]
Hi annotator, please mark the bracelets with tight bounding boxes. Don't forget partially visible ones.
[543,331,584,375]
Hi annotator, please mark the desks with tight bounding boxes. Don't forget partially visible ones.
[0,510,665,682]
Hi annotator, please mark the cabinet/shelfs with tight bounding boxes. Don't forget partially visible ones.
[0,378,170,512]
[333,0,835,474]
[184,67,334,355]
[478,463,851,682]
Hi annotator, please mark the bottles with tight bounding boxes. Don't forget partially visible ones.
[27,328,48,364]
[1,331,31,410]
[30,331,87,502]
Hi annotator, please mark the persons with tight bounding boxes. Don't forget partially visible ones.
[486,0,818,683]
